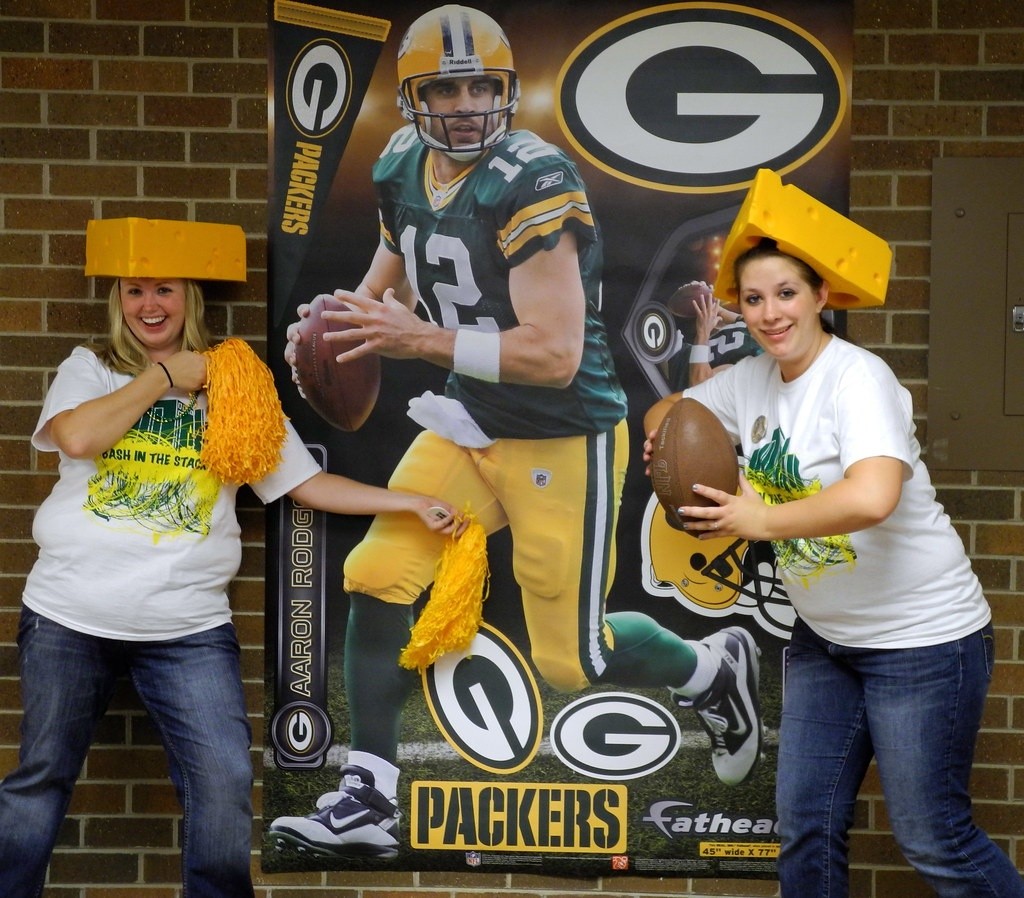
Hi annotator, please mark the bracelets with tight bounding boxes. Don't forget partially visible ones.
[156,360,174,391]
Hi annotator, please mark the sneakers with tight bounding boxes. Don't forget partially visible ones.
[671,625,768,787]
[268,763,405,865]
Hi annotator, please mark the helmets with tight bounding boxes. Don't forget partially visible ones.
[397,4,517,132]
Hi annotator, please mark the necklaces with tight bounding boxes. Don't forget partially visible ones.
[145,391,199,423]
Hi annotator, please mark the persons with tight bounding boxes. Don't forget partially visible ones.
[262,2,765,869]
[1,274,474,898]
[665,281,767,390]
[646,246,1023,896]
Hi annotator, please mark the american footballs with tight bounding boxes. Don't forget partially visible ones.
[294,290,383,436]
[668,286,715,319]
[649,396,740,541]
